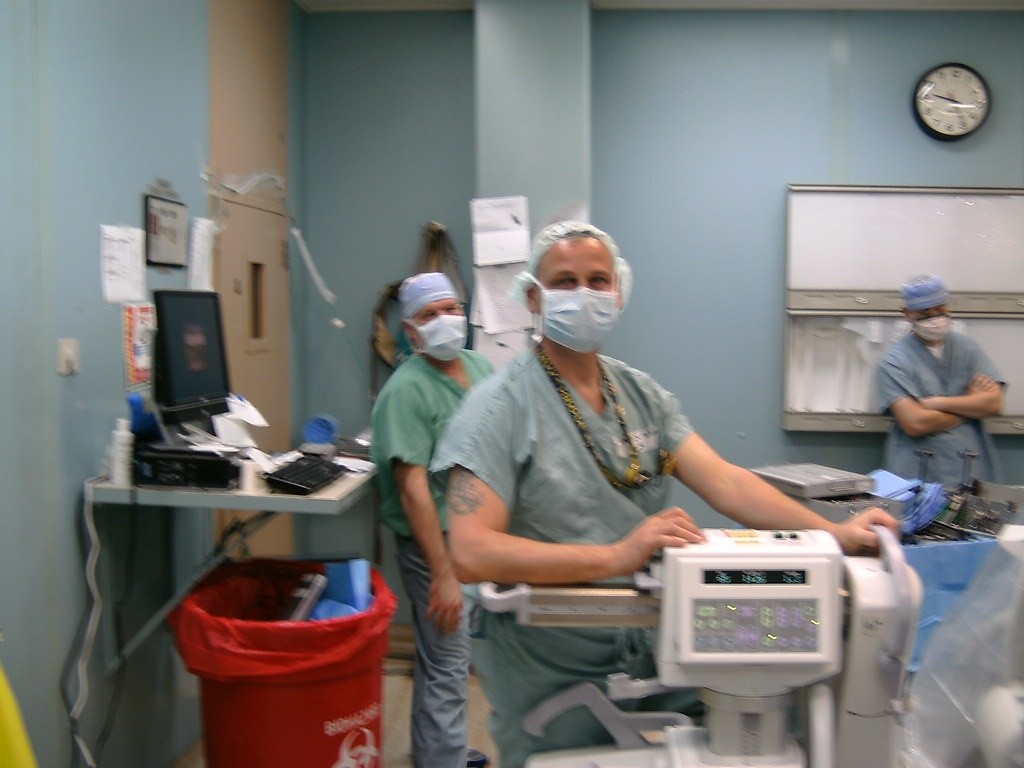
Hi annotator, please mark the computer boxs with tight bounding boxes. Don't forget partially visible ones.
[131,445,249,490]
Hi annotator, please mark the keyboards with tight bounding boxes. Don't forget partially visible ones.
[266,455,348,494]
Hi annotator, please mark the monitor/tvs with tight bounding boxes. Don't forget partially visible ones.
[153,290,232,437]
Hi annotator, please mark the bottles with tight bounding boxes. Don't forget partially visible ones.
[109,418,134,487]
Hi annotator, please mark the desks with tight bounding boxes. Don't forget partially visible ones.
[83,453,376,673]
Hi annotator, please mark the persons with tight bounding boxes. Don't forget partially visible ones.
[367,271,500,768]
[446,218,903,768]
[876,271,1008,487]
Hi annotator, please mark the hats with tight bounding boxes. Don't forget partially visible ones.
[398,272,458,318]
[901,274,950,311]
[528,220,620,276]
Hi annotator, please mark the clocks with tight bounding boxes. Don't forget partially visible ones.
[912,61,993,142]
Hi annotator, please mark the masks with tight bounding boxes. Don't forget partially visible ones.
[532,280,619,353]
[909,315,951,342]
[405,315,468,361]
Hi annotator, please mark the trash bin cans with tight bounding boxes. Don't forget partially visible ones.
[177,555,398,768]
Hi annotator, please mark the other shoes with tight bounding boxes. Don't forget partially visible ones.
[467,747,488,768]
